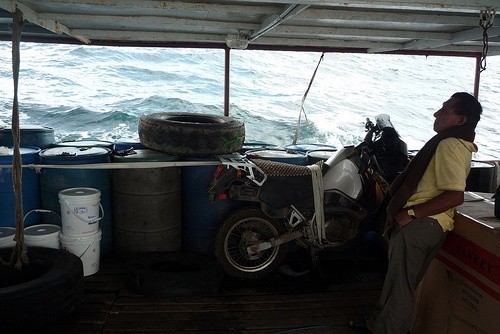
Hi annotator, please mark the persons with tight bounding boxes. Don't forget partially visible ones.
[348,92,482,334]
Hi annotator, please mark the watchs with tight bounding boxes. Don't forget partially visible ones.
[406,205,418,220]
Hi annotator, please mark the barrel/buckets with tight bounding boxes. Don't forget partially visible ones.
[0,127,500,277]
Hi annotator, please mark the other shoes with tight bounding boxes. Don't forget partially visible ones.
[348,319,370,332]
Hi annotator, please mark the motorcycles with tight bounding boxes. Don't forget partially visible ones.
[207,112,414,282]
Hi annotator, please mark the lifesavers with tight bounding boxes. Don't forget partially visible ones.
[138,111,246,154]
